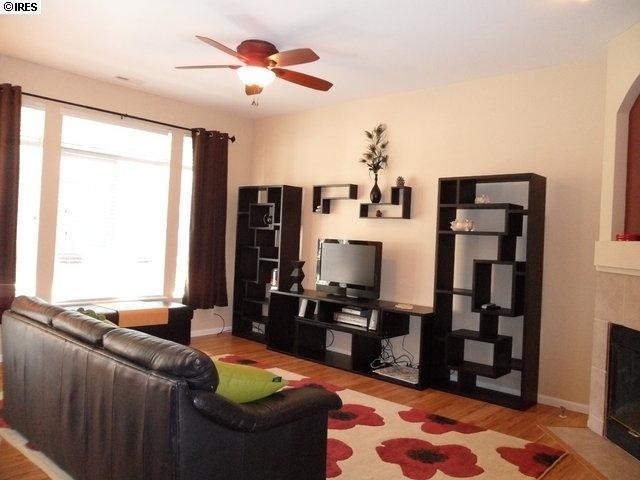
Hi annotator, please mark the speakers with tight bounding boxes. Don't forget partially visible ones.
[298,298,316,318]
[368,309,378,331]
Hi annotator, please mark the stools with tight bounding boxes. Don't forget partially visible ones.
[63,301,193,345]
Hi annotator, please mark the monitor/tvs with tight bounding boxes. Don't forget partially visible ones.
[316,239,383,302]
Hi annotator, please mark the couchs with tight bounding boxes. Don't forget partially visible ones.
[1,295,343,480]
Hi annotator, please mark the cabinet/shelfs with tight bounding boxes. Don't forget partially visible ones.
[231,185,303,343]
[268,288,434,392]
[432,173,546,411]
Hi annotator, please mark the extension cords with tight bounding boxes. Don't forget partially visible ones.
[400,364,419,372]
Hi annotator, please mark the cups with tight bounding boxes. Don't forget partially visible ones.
[258,190,268,203]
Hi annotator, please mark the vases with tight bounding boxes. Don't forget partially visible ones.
[370,173,382,203]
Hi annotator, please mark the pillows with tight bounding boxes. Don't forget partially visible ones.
[213,359,289,404]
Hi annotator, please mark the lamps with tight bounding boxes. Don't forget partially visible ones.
[237,64,277,106]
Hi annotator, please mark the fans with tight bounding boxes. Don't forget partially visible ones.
[173,35,334,92]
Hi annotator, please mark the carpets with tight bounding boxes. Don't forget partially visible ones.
[0,353,570,480]
